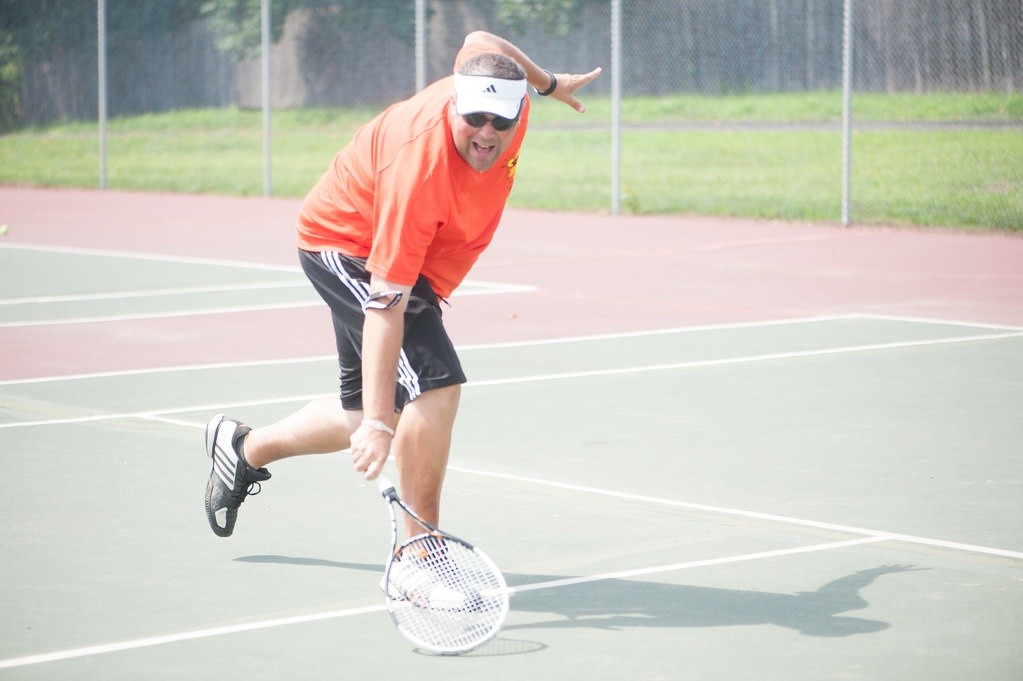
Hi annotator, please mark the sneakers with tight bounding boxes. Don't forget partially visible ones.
[205,412,272,537]
[380,549,482,611]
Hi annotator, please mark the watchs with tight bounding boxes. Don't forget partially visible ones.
[360,418,395,436]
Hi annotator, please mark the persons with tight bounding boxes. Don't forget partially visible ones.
[203,30,602,613]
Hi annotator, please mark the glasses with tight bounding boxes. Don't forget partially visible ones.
[463,98,524,131]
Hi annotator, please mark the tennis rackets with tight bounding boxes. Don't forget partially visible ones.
[374,463,510,653]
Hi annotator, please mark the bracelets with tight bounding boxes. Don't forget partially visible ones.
[534,69,557,96]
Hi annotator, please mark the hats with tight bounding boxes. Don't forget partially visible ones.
[453,72,527,120]
